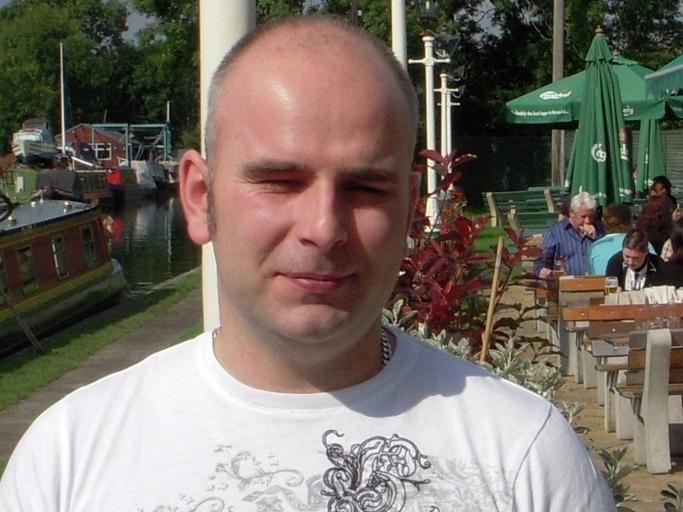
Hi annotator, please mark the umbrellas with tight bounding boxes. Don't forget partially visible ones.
[565,28,637,208]
[503,52,682,126]
[644,50,682,101]
[637,111,667,191]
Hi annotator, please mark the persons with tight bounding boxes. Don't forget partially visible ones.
[534,175,682,294]
[0,12,619,512]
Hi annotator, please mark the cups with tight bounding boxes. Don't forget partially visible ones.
[603,276,618,296]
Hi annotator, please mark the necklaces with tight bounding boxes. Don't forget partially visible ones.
[370,319,393,373]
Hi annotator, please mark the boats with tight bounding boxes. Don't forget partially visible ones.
[0,194,131,356]
[0,41,181,207]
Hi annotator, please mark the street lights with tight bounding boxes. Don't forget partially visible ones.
[403,0,467,241]
